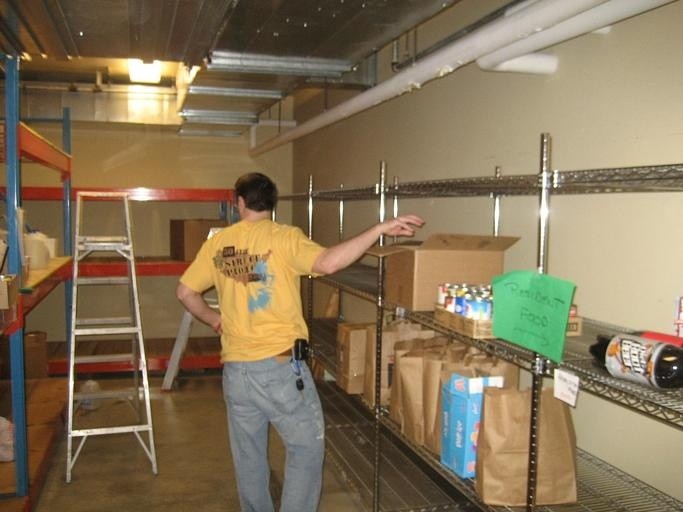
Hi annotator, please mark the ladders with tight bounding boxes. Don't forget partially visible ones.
[66,191,157,483]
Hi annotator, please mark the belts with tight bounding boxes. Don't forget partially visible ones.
[276,348,296,358]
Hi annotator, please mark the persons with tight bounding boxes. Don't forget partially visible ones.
[176,172,426,512]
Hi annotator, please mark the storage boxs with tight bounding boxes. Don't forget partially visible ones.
[336,321,376,396]
[1,332,47,377]
[171,219,230,263]
[363,232,521,313]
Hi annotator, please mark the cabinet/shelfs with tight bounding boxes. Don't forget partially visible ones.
[272,133,683,511]
[1,187,235,375]
[0,58,73,512]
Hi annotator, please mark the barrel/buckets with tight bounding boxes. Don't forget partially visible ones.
[78,372,102,412]
[23,330,49,379]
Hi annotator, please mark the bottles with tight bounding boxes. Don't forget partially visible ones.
[586,329,682,392]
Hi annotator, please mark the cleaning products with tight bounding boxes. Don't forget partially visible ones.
[438,281,491,320]
